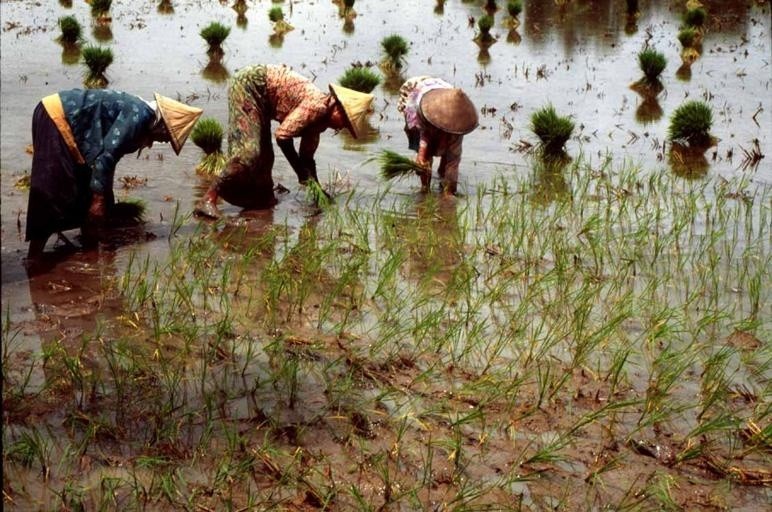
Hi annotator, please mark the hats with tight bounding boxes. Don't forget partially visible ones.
[420,88,479,136]
[329,83,374,138]
[152,92,203,155]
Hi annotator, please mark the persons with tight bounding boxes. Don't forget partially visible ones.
[194,64,374,220]
[23,86,204,259]
[396,75,479,201]
